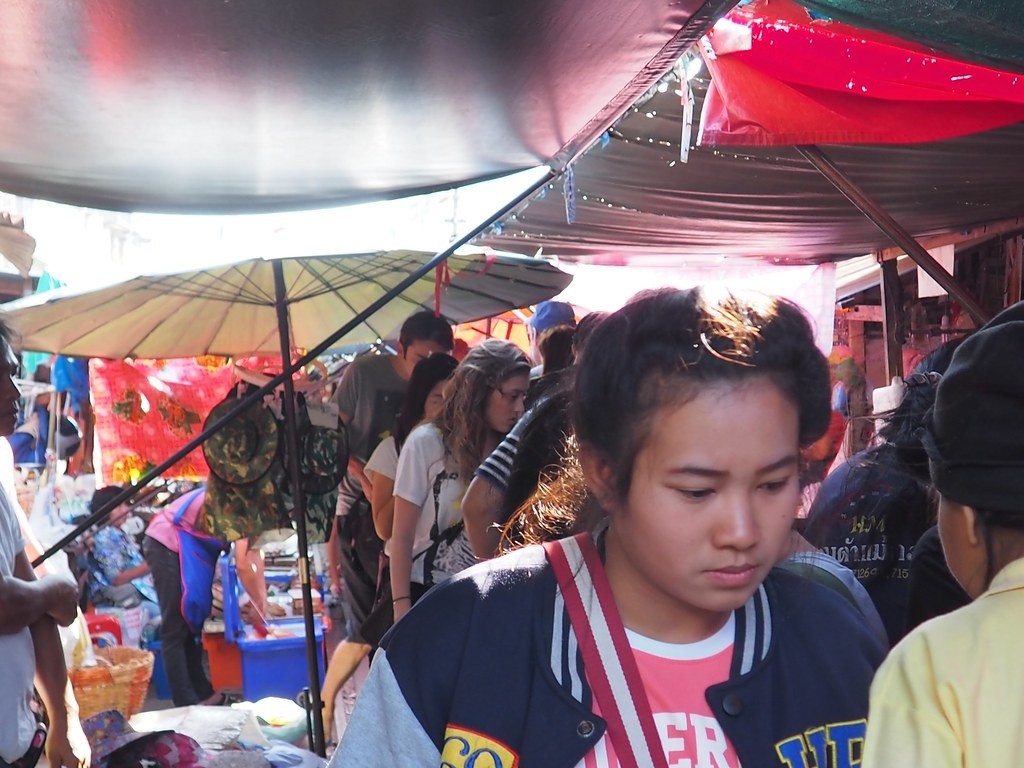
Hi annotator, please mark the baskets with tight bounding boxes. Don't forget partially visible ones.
[69,635,154,721]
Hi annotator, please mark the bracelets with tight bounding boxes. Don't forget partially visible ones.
[392,596,412,603]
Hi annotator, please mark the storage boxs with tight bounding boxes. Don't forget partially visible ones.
[220,554,326,707]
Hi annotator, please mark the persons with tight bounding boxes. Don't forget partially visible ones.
[0,317,268,768]
[799,299,1024,768]
[327,280,888,768]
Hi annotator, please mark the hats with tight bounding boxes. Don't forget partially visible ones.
[913,300,1024,513]
[529,301,576,335]
[201,393,280,485]
[81,709,272,768]
[280,401,348,496]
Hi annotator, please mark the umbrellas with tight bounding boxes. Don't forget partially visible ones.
[0,247,574,759]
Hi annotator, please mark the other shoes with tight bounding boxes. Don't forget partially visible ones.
[202,693,242,706]
[322,718,333,760]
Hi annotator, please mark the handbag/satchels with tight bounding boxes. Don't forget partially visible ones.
[101,582,141,610]
